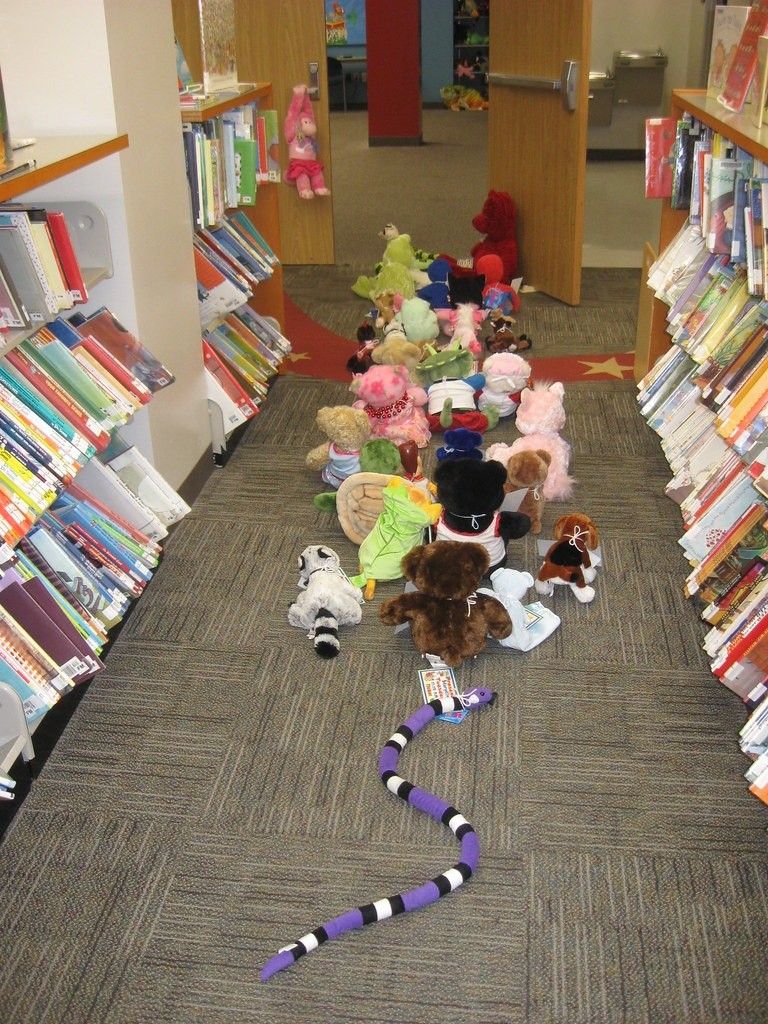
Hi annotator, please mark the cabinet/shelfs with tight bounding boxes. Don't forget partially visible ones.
[1,134,165,839]
[180,82,289,468]
[455,15,489,74]
[646,89,768,377]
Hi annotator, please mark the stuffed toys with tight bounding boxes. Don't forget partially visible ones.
[379,541,512,667]
[288,544,362,657]
[535,513,599,602]
[305,406,371,490]
[440,85,487,110]
[257,688,497,979]
[466,34,489,44]
[476,568,535,648]
[313,190,572,599]
[457,64,474,78]
[284,84,332,199]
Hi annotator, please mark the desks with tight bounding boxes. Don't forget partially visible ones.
[337,55,367,74]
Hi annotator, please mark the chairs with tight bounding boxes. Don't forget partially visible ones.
[327,56,347,113]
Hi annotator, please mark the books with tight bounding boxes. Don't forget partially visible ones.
[634,1,767,800]
[175,35,294,430]
[1,203,192,716]
[10,137,35,150]
